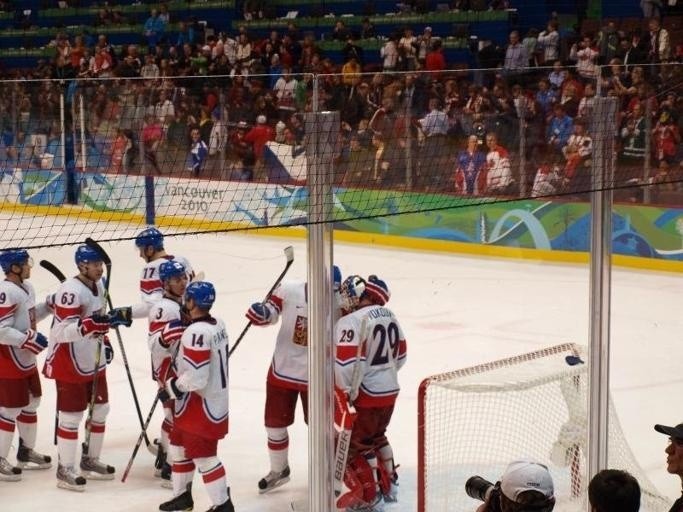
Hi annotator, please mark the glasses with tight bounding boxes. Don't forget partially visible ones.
[668,438,683,448]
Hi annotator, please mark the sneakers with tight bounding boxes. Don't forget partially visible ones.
[17,446,51,464]
[206,499,233,512]
[345,493,384,511]
[259,466,289,489]
[56,463,86,485]
[80,456,114,474]
[0,457,22,475]
[160,493,193,512]
[161,464,172,481]
[388,481,399,497]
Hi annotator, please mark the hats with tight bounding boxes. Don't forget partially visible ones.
[655,424,683,441]
[500,461,554,506]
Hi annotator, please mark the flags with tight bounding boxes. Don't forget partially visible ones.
[263,140,307,186]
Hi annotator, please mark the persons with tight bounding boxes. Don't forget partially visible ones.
[473,459,557,512]
[333,273,408,511]
[337,274,368,316]
[655,421,683,511]
[0,246,55,477]
[42,244,134,487]
[0,0,683,210]
[108,227,207,327]
[159,281,235,512]
[587,468,641,511]
[147,259,190,483]
[247,264,342,492]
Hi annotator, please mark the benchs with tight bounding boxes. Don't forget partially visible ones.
[0,0,683,208]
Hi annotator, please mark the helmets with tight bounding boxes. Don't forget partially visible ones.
[0,248,29,273]
[159,261,186,282]
[186,282,215,309]
[75,245,104,267]
[334,266,341,291]
[340,276,366,314]
[136,228,163,249]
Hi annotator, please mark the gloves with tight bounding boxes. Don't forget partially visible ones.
[17,329,48,355]
[246,303,271,326]
[108,308,131,328]
[158,377,184,404]
[46,294,56,312]
[162,320,191,346]
[81,315,111,339]
[364,275,390,305]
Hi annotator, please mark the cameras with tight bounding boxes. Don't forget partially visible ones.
[465,475,501,506]
